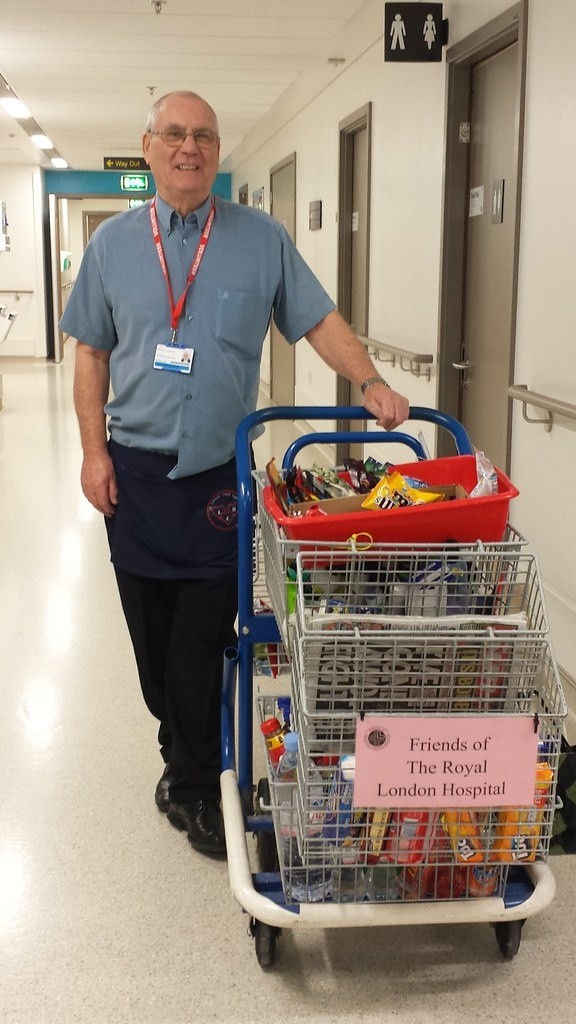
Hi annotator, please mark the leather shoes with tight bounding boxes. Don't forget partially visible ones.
[166,795,226,852]
[154,749,209,812]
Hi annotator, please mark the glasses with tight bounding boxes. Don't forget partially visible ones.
[148,126,219,147]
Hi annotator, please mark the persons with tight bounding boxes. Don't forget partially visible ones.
[58,90,410,859]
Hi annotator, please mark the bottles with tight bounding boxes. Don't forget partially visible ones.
[277,695,299,733]
[276,733,332,902]
[260,717,292,777]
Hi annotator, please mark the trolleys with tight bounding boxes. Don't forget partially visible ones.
[217,405,569,966]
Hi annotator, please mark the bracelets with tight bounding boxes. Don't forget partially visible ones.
[361,377,390,394]
